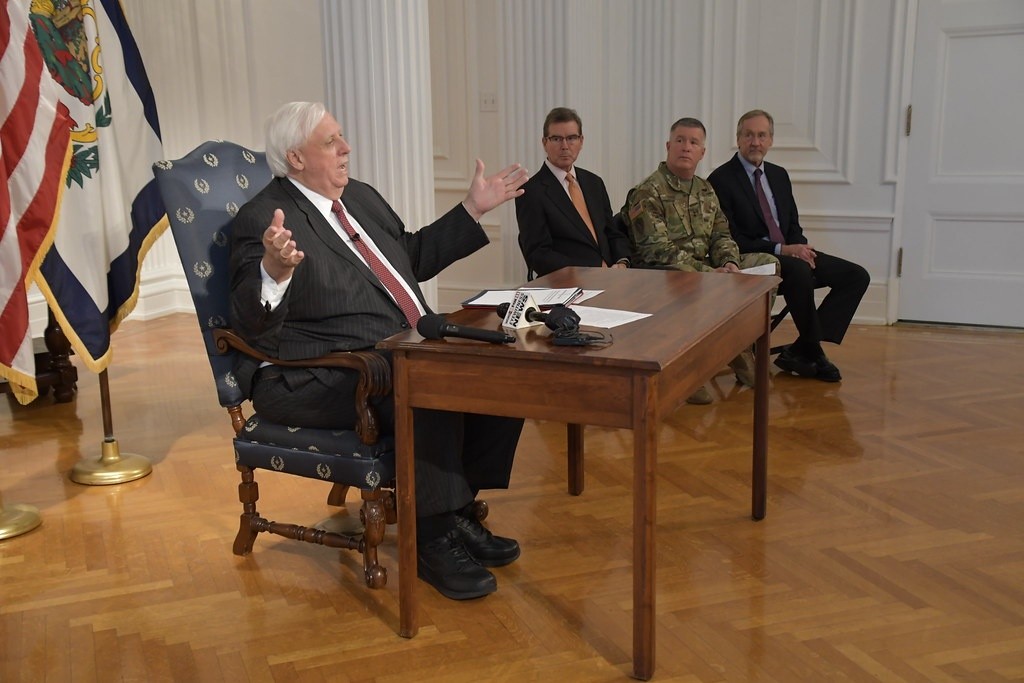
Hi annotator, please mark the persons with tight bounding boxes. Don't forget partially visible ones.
[629,118,780,404]
[513,108,632,278]
[706,110,872,383]
[209,101,528,604]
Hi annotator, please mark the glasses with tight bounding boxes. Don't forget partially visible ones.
[545,135,580,144]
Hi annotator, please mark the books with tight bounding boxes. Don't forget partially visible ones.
[462,285,584,310]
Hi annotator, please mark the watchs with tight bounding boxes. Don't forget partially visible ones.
[617,260,630,268]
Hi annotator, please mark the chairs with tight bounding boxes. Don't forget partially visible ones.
[517,226,829,387]
[151,140,487,588]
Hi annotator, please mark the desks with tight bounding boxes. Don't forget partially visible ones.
[374,268,783,683]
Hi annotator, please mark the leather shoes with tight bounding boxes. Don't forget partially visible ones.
[417,525,497,601]
[456,516,520,567]
[774,348,842,382]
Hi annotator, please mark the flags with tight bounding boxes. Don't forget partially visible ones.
[26,0,176,374]
[0,0,73,408]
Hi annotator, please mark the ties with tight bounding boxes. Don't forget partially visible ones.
[331,198,422,330]
[754,168,787,248]
[565,172,598,245]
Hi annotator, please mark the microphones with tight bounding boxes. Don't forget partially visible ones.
[496,291,581,334]
[417,314,516,345]
[354,233,360,239]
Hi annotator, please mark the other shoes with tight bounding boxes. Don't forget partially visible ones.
[727,349,756,387]
[686,387,714,405]
